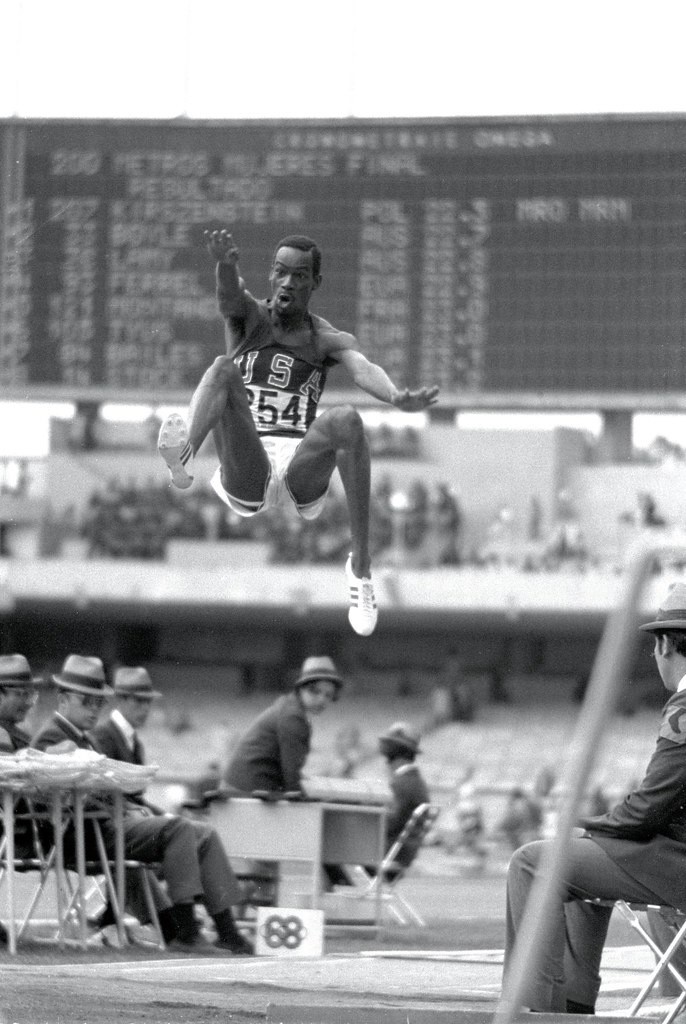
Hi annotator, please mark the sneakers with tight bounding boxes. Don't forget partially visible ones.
[345,551,378,638]
[157,412,195,489]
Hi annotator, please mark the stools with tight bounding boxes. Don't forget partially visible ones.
[0,811,166,952]
[581,897,686,1024]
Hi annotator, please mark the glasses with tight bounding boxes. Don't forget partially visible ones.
[0,686,40,706]
[63,689,109,709]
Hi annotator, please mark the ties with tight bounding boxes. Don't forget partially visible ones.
[133,733,141,766]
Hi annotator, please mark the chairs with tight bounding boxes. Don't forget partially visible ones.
[342,803,441,927]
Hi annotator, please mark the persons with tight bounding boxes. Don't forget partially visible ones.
[500,583,685,1015]
[0,646,432,955]
[158,228,440,638]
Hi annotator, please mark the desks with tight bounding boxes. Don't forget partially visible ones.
[180,797,390,939]
[0,780,143,955]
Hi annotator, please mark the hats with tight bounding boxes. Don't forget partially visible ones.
[51,655,114,696]
[377,721,423,754]
[109,666,162,698]
[637,582,686,633]
[0,653,43,685]
[291,656,343,690]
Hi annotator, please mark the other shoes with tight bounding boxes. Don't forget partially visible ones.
[215,929,256,955]
[531,991,598,1014]
[173,933,231,956]
